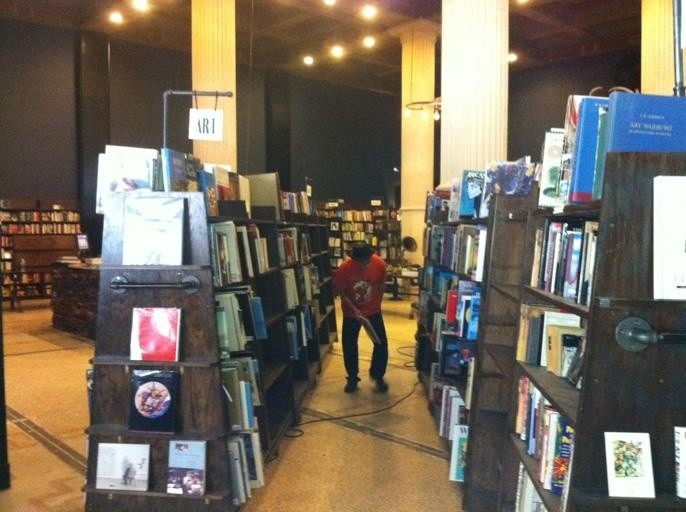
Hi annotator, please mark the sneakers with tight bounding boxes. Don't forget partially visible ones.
[344,382,356,392]
[375,378,386,392]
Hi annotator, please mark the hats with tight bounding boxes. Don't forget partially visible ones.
[346,242,374,261]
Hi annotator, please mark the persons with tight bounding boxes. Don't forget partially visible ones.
[331,238,391,393]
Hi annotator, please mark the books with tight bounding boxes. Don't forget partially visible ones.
[94,145,207,497]
[416,92,685,512]
[1,209,82,297]
[193,158,330,506]
[316,204,401,289]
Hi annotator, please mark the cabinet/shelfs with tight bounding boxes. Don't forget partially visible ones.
[314,205,400,303]
[80,192,339,511]
[0,197,81,301]
[415,152,685,512]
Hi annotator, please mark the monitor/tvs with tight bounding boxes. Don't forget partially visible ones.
[75,233,90,257]
